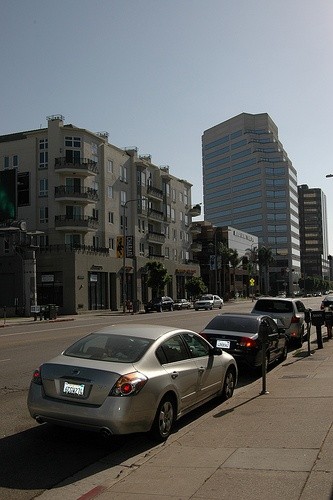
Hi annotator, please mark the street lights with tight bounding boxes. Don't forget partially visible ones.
[122,197,148,311]
[251,242,265,302]
[214,228,231,295]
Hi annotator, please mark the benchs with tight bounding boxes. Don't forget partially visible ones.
[86,347,137,360]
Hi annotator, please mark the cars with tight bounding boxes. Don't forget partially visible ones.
[320,297,333,311]
[173,299,191,311]
[250,298,311,347]
[199,313,288,374]
[27,325,238,441]
[194,295,223,311]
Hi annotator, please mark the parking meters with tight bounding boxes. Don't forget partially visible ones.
[257,319,270,395]
[304,308,312,355]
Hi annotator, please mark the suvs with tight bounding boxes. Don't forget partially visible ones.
[144,296,174,313]
